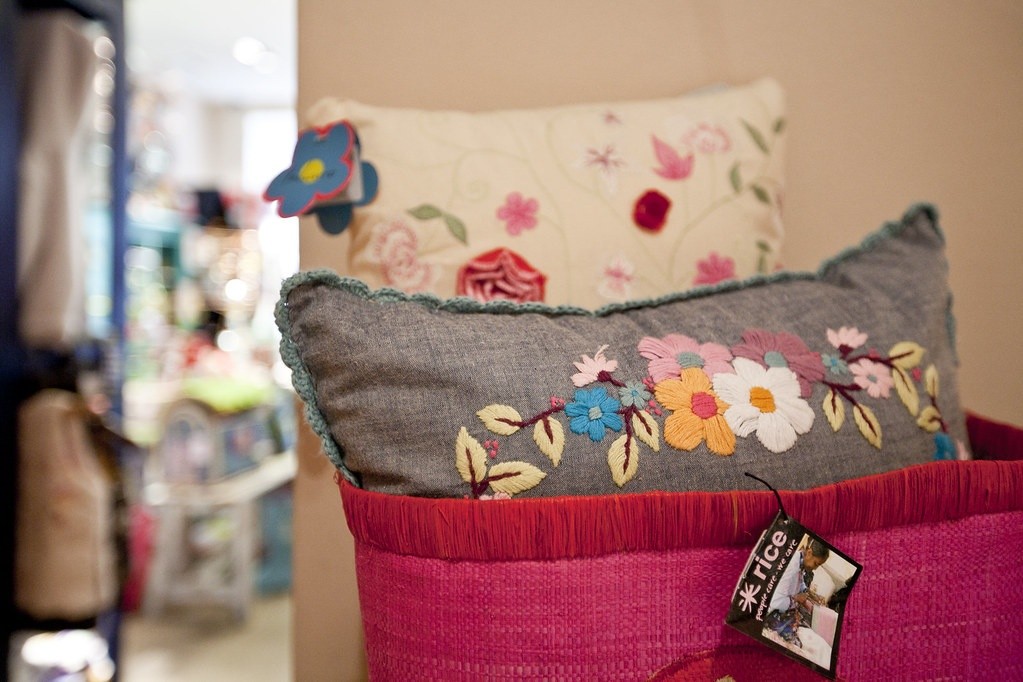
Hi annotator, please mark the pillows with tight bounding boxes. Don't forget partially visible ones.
[301,70,790,309]
[273,200,973,502]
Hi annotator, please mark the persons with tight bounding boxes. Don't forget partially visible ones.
[766,539,829,649]
